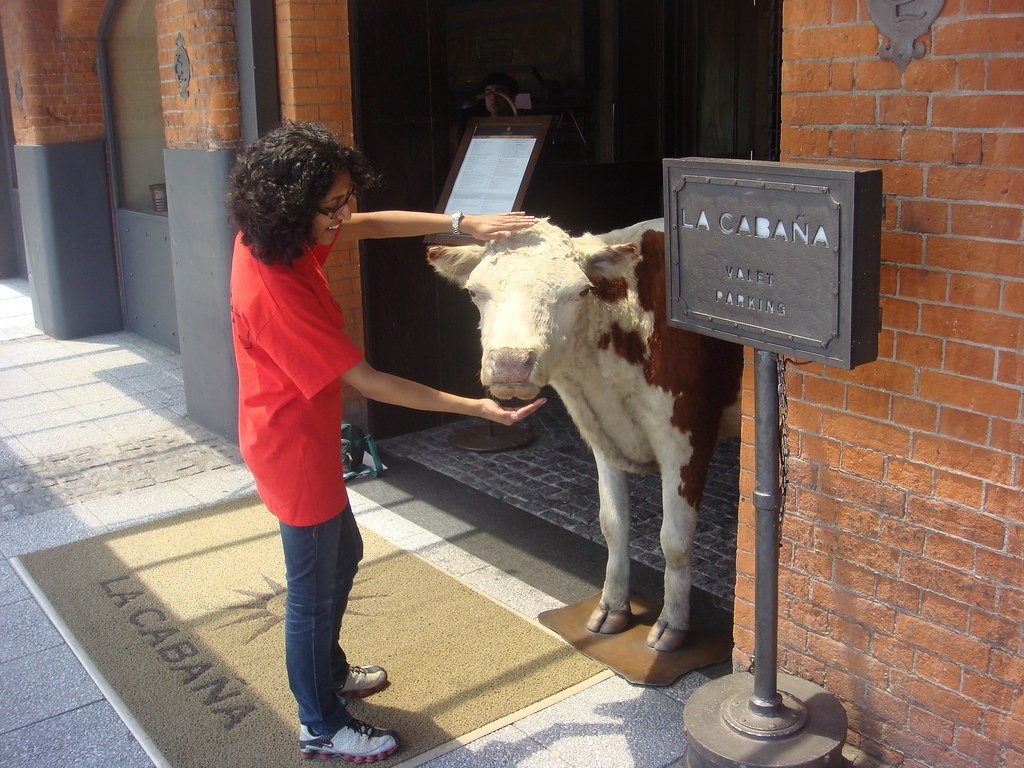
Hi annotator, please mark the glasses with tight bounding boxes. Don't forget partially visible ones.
[317,181,356,220]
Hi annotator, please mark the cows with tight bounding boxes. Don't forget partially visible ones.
[425,214,744,653]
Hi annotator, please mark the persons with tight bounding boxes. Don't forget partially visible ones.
[481,73,518,117]
[230,122,547,763]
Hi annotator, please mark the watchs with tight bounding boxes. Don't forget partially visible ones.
[452,211,463,235]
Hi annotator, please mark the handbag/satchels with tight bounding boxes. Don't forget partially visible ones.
[340,420,382,480]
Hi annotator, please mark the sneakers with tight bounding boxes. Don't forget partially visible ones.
[335,665,387,699]
[299,710,401,764]
